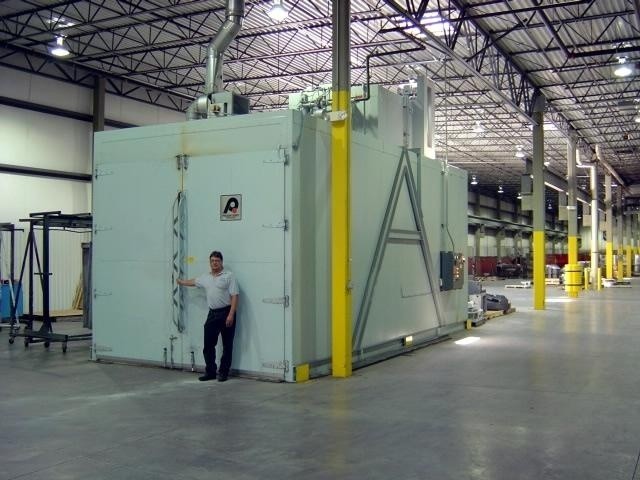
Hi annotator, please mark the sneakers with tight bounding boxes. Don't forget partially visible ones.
[198,375,216,381]
[218,375,227,382]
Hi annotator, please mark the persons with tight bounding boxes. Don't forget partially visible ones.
[176,251,240,382]
